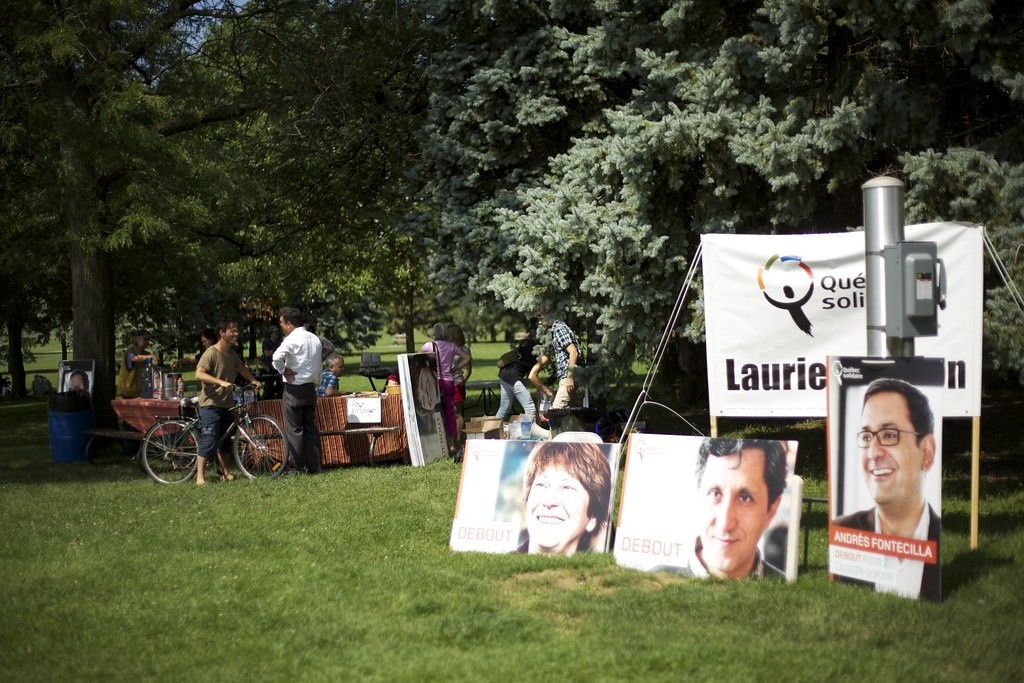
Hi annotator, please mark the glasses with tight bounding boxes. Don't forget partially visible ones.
[856,428,921,448]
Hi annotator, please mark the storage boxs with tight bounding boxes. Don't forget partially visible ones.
[458,414,521,438]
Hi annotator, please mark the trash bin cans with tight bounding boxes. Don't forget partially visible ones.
[47,390,97,462]
[543,409,601,439]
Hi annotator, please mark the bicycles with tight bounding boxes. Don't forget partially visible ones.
[138,382,290,487]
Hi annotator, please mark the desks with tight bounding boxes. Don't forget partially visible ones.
[114,393,411,468]
[359,367,399,391]
[247,372,285,400]
[462,380,519,417]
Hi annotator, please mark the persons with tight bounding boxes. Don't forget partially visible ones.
[69,369,89,392]
[418,324,472,454]
[316,353,346,398]
[496,339,555,422]
[649,438,787,583]
[169,329,217,368]
[272,307,323,478]
[534,305,586,408]
[507,442,611,556]
[832,379,941,603]
[195,320,261,485]
[318,336,335,358]
[32,375,50,394]
[261,326,282,399]
[117,330,161,457]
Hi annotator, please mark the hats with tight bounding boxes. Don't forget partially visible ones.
[268,326,280,333]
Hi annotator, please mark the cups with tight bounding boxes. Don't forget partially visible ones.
[188,386,192,397]
[193,386,197,397]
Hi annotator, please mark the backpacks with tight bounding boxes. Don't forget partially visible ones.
[497,349,519,368]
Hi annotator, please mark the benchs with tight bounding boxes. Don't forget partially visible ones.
[232,425,399,468]
[79,429,160,470]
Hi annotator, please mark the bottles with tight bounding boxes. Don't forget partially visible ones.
[176,378,183,395]
[179,382,184,397]
[386,375,396,385]
[141,358,160,398]
